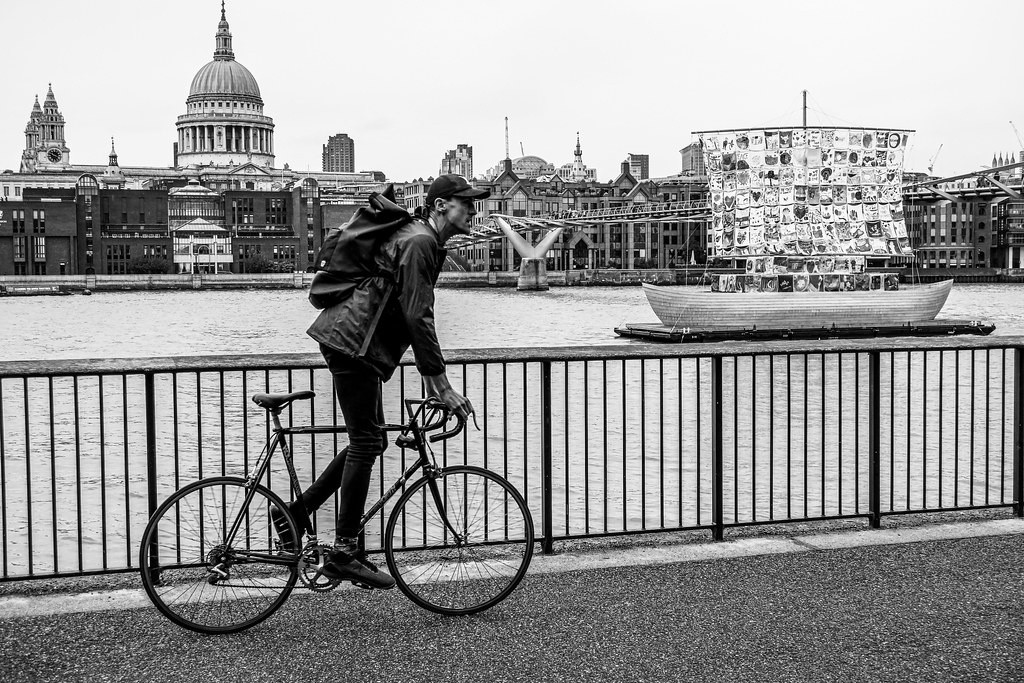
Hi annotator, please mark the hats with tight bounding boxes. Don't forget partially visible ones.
[425,173,491,204]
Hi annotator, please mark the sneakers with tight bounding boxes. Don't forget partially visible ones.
[322,546,396,590]
[269,502,306,554]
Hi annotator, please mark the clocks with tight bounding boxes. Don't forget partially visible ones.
[47,148,62,163]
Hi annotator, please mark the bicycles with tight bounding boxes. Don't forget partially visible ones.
[137,388,536,637]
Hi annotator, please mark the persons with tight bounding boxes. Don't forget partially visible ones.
[266,175,493,591]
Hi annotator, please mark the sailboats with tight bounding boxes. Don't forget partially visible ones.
[636,122,957,331]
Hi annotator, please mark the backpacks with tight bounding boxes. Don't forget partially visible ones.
[308,184,441,311]
